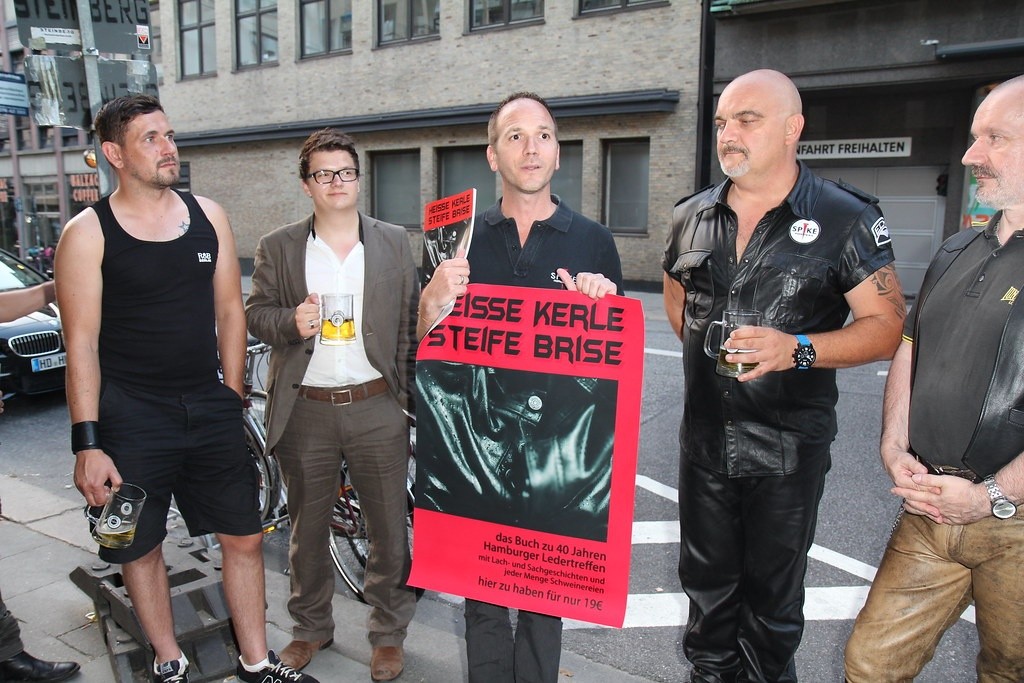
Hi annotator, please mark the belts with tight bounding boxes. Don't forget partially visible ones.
[297,377,391,406]
[910,448,982,485]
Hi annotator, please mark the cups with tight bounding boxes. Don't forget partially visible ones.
[84,484,147,550]
[313,293,357,346]
[704,309,764,378]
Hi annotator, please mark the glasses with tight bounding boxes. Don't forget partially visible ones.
[305,168,359,184]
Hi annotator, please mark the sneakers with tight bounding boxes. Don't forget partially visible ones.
[153,648,189,683]
[238,650,319,683]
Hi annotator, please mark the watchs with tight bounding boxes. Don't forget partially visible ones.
[984,474,1017,519]
[792,334,816,370]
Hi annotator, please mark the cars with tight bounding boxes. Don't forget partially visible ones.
[0,247,67,403]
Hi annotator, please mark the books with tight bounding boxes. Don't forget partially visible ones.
[422,187,476,293]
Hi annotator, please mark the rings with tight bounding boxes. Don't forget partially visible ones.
[309,320,314,328]
[459,276,464,284]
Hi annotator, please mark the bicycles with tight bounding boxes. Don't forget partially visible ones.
[218,334,428,611]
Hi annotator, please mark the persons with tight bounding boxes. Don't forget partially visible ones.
[0,281,81,683]
[843,75,1024,683]
[661,68,906,683]
[244,126,420,683]
[54,94,320,683]
[416,93,622,683]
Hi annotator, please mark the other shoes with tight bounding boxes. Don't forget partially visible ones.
[370,646,405,682]
[276,638,333,672]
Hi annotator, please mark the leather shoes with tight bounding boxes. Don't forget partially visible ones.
[0,651,80,683]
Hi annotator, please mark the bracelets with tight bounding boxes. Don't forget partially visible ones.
[418,312,434,324]
[72,420,100,454]
